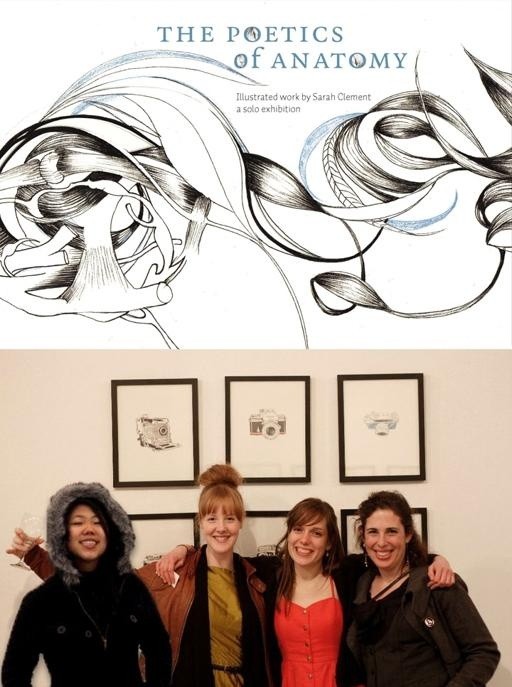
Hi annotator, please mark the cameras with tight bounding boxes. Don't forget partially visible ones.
[367,413,400,435]
[136,417,177,449]
[249,409,286,439]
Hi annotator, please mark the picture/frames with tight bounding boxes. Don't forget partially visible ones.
[108,372,429,588]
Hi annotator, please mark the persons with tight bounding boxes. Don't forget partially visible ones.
[7,464,273,687]
[155,497,455,686]
[1,482,172,686]
[343,491,501,687]
